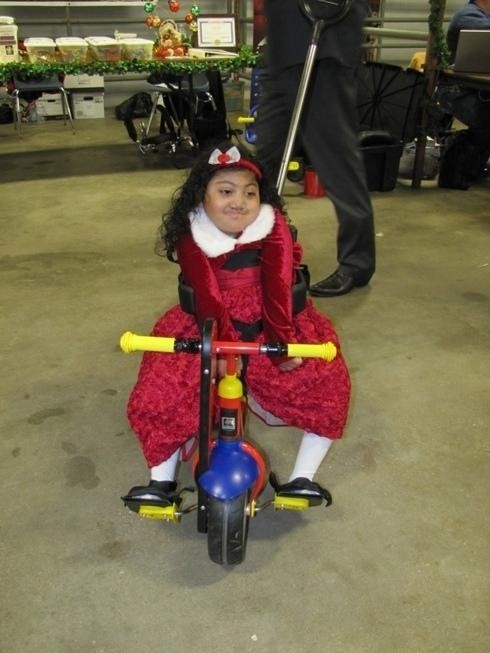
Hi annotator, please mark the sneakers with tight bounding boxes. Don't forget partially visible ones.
[278,476,322,507]
[120,480,177,513]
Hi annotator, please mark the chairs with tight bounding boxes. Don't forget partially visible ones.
[13,69,74,136]
[115,70,225,169]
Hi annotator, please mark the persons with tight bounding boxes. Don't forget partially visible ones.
[127,142,352,508]
[446,0,490,64]
[253,0,375,299]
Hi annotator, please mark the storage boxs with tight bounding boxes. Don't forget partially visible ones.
[24,36,155,63]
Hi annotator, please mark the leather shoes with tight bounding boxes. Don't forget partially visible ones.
[309,269,356,297]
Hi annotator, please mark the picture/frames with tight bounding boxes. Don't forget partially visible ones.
[194,14,241,52]
